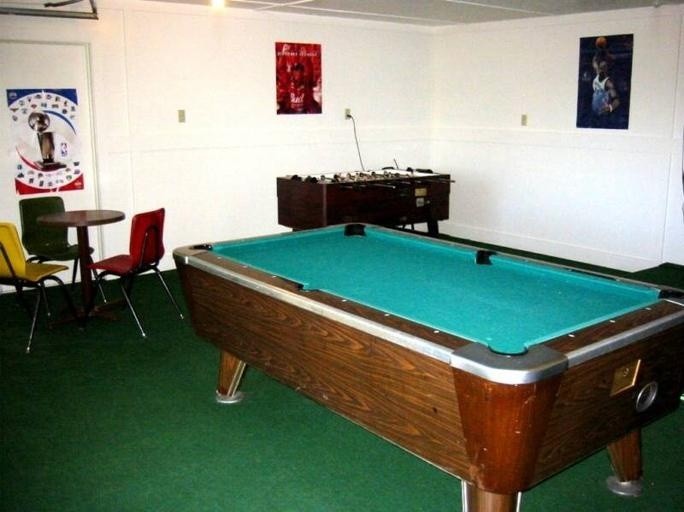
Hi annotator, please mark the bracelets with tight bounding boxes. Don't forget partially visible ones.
[608,104,612,113]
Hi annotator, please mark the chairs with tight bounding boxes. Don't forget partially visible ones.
[0,224,78,354]
[16,197,108,316]
[86,208,184,337]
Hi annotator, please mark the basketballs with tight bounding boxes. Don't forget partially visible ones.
[596,38,607,49]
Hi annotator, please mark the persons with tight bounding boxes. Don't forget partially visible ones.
[583,43,621,124]
[276,44,321,114]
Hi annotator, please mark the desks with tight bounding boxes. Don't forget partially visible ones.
[173,217,684,511]
[36,211,124,329]
